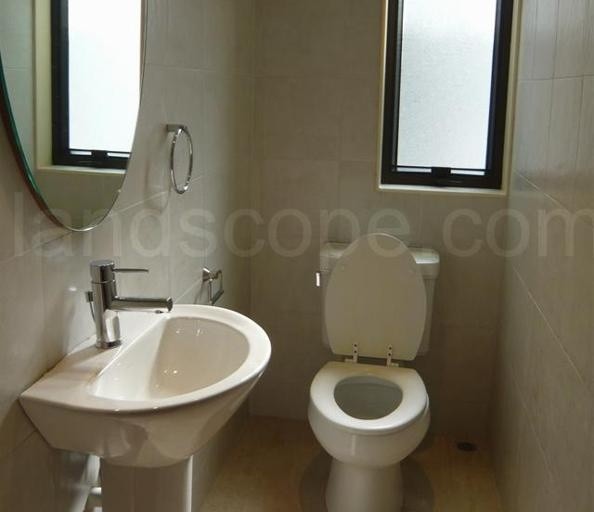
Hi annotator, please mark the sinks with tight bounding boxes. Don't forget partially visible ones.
[18,304,272,467]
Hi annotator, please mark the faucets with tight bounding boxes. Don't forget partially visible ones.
[86,258,175,348]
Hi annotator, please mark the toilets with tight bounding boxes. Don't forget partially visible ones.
[309,233,441,511]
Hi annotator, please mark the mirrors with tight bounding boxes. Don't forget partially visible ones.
[0,2,147,235]
